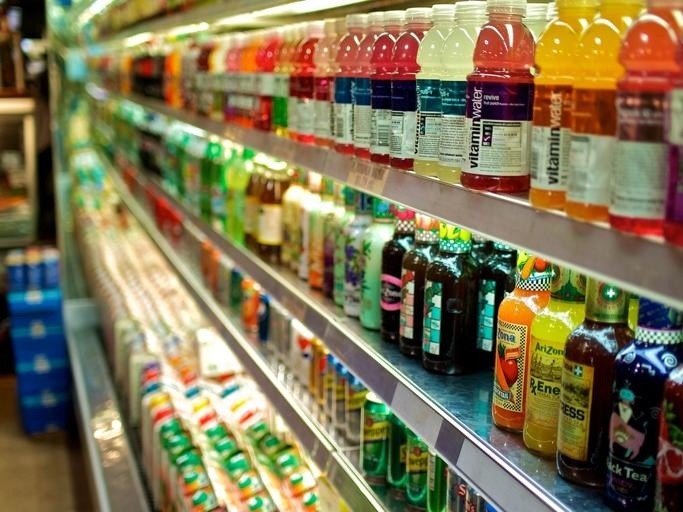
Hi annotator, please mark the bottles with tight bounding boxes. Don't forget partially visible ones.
[86,2,682,510]
[9,242,63,284]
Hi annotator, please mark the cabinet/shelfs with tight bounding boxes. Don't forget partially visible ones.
[52,0,683,512]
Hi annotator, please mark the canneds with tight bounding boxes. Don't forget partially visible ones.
[199,241,497,512]
